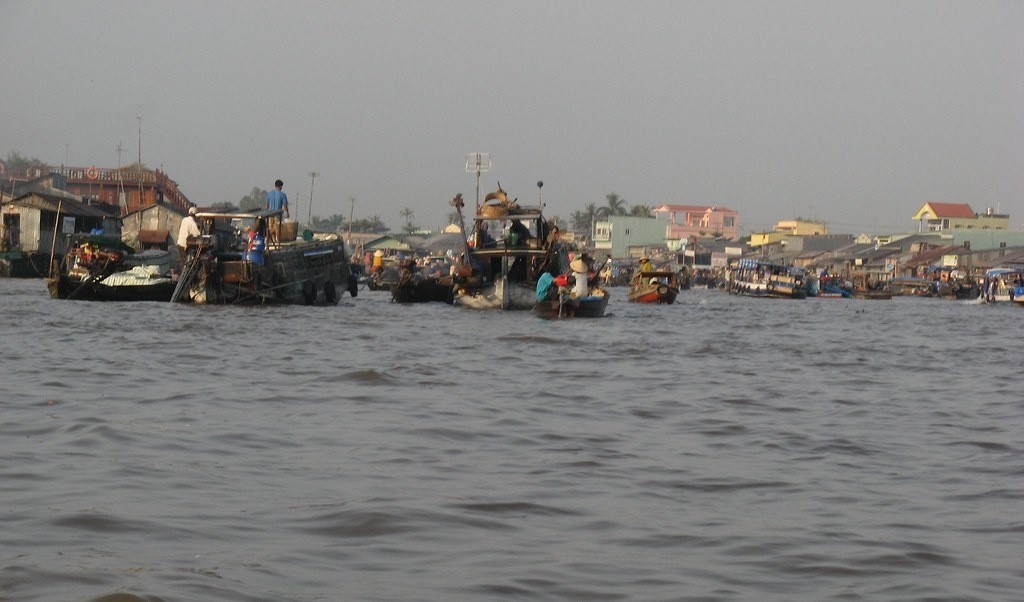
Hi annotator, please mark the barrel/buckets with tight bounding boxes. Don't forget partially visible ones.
[248,232,264,265]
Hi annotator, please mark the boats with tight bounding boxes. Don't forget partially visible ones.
[46,153,1023,319]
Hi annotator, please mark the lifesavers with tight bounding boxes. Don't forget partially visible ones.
[766,283,775,293]
[658,284,669,296]
[87,168,99,179]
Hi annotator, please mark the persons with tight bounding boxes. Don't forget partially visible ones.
[834,273,840,285]
[820,269,829,290]
[604,266,628,287]
[371,250,384,277]
[725,268,732,287]
[985,277,998,296]
[568,259,588,297]
[265,180,290,243]
[639,256,652,285]
[536,263,559,303]
[547,226,559,243]
[177,207,200,271]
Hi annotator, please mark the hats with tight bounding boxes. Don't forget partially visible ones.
[189,206,198,215]
[639,256,649,263]
[569,259,588,273]
[374,250,384,256]
